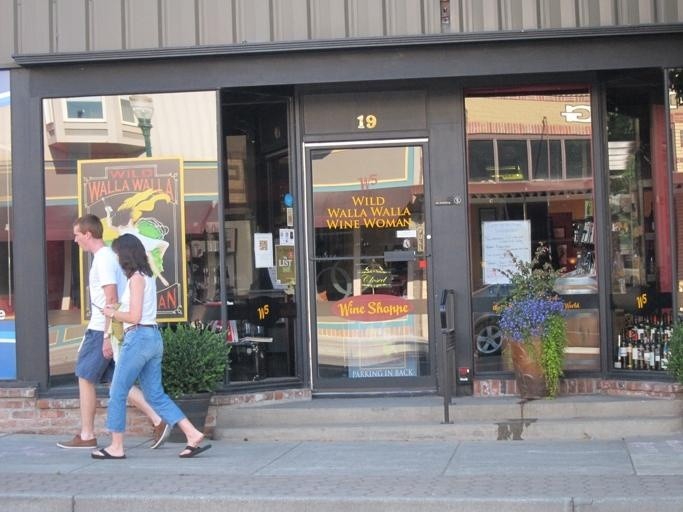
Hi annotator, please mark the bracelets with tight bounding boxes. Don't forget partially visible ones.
[110,310,115,318]
[103,333,110,339]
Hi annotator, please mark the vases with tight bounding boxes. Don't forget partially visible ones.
[513,321,555,398]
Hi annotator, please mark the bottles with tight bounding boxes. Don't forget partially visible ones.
[615,310,674,370]
[102,198,115,217]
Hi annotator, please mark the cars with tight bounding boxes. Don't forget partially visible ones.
[473,283,520,355]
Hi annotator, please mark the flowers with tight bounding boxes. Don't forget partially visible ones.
[492,243,565,398]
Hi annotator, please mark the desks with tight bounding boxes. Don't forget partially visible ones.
[207,300,296,375]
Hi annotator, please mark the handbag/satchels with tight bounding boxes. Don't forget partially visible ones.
[112,305,123,343]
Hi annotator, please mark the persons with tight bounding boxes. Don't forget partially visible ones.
[90,233,213,459]
[55,213,172,449]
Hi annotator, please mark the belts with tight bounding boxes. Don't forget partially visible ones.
[124,323,160,334]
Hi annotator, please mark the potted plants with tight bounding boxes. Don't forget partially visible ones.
[153,323,233,442]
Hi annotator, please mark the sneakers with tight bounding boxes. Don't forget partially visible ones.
[56,434,97,448]
[150,418,169,449]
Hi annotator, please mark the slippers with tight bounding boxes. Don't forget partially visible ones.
[179,445,212,458]
[91,448,126,458]
